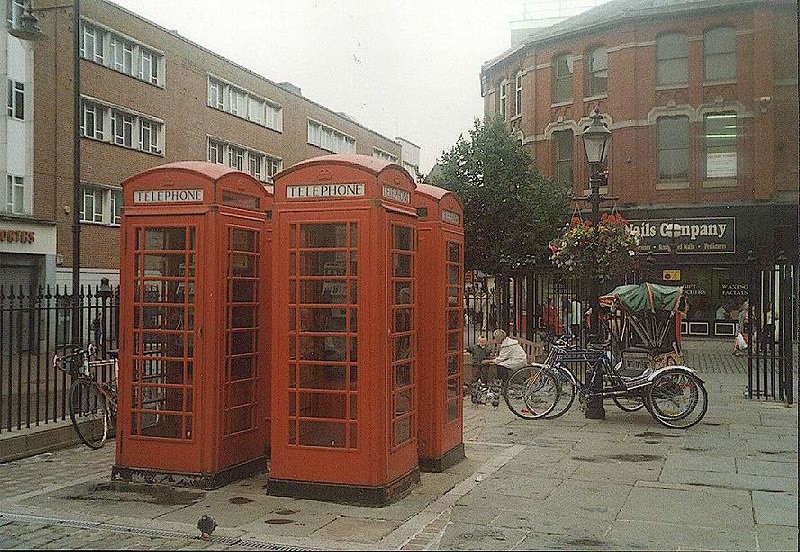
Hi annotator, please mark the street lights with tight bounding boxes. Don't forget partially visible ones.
[8,1,81,404]
[559,100,620,421]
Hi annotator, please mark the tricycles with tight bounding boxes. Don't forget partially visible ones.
[503,281,710,428]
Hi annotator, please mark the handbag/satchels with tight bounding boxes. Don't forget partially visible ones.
[738,332,748,350]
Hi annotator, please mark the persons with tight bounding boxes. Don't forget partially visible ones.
[677,293,780,358]
[541,294,609,344]
[464,334,491,391]
[91,310,104,357]
[482,329,527,394]
[460,286,516,328]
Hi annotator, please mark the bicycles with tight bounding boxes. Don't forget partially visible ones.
[52,340,160,449]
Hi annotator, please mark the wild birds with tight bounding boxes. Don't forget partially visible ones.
[197,514,219,542]
[462,378,514,409]
